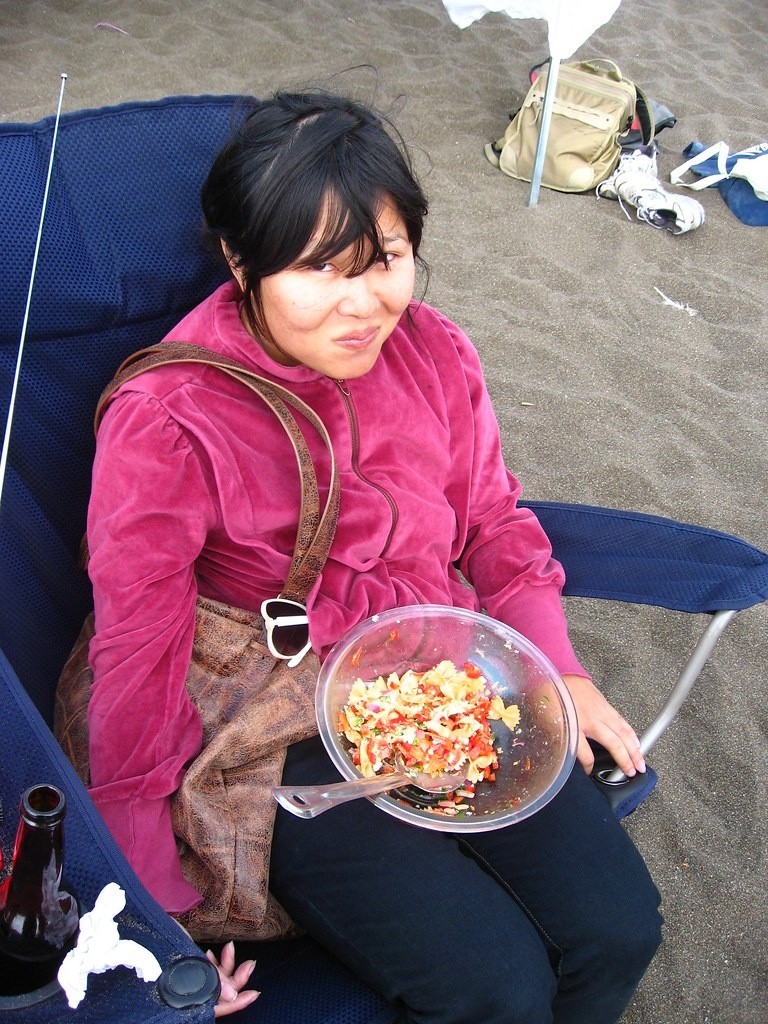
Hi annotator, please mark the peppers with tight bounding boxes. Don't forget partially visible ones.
[338,662,500,805]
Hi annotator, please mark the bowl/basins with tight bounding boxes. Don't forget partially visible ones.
[315,605,579,833]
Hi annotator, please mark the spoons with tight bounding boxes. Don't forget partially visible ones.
[271,747,471,819]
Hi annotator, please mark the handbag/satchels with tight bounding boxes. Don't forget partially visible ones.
[669,140,768,226]
[484,58,656,193]
[510,58,676,157]
[53,341,338,944]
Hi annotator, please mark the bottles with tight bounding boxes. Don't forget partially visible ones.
[0,782,82,997]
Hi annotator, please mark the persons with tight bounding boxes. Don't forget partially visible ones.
[85,90,664,1024]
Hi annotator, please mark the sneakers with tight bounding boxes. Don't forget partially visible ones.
[596,148,657,220]
[614,170,706,235]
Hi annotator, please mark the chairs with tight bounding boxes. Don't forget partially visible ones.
[1,97,768,1023]
